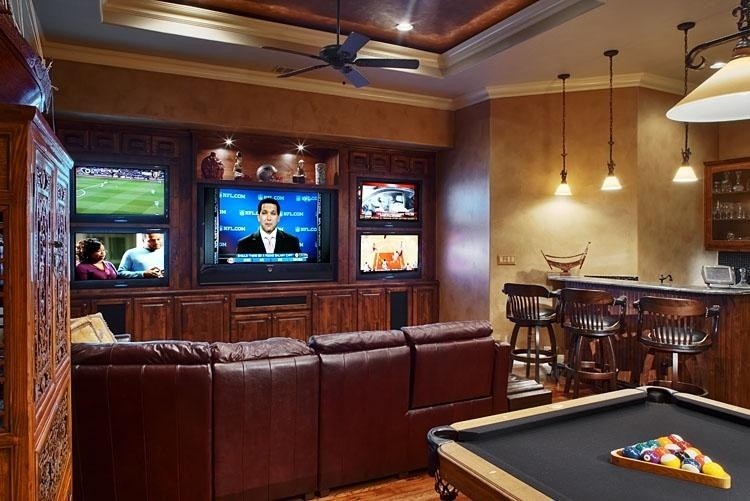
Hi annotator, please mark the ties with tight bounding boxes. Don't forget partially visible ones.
[265,237,274,254]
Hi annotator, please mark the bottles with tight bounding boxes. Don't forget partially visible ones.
[202,152,225,180]
[735,269,750,287]
[713,170,747,221]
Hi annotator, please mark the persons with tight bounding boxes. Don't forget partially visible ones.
[235,198,302,254]
[364,242,414,272]
[117,233,164,279]
[75,238,118,281]
[77,167,164,210]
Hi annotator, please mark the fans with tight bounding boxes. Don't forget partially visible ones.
[261,0,419,88]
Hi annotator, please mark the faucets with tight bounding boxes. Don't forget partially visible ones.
[658,274,673,284]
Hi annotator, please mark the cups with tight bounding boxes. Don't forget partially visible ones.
[292,176,306,184]
[315,164,327,187]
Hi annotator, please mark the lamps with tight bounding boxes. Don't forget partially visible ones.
[554,74,572,195]
[666,0,750,123]
[672,22,699,183]
[601,49,622,190]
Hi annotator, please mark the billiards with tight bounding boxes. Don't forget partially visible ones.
[622,434,724,478]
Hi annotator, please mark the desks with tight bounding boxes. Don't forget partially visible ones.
[427,386,750,501]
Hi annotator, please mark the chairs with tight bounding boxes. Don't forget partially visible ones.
[501,283,721,400]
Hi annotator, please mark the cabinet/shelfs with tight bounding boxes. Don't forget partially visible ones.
[703,156,750,252]
[0,103,74,501]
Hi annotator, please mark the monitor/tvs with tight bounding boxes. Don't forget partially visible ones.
[355,228,422,279]
[355,177,422,229]
[702,265,734,285]
[197,181,338,283]
[69,227,170,287]
[70,160,170,224]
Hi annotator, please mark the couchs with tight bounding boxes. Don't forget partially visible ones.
[71,320,511,501]
